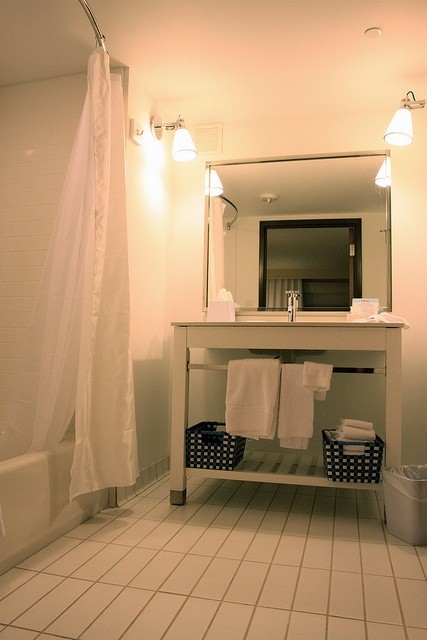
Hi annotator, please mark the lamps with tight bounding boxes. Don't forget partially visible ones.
[383,91,426,146]
[205,170,238,230]
[151,114,198,162]
[375,157,392,190]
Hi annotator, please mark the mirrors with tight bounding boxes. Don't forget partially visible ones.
[202,149,392,314]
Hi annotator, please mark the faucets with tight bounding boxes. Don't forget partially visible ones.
[285,290,299,322]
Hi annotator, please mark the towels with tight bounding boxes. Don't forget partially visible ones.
[278,364,313,450]
[226,359,280,441]
[302,360,333,402]
[353,314,409,329]
[324,418,379,483]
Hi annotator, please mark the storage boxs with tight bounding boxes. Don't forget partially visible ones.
[185,421,246,471]
[321,430,385,484]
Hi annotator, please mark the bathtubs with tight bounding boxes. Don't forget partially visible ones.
[0,434,110,577]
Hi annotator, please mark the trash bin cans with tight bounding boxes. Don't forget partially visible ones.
[378,463,427,547]
